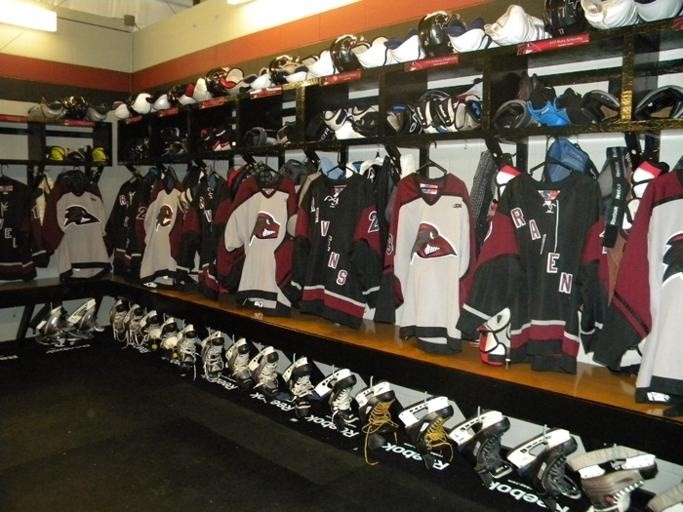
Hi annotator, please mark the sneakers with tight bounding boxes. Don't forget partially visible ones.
[38,307,96,347]
[327,374,357,433]
[456,416,515,489]
[404,404,453,471]
[228,344,253,392]
[251,351,280,402]
[515,435,583,512]
[177,330,198,368]
[284,364,315,419]
[358,390,402,450]
[201,337,225,380]
[109,304,176,358]
[580,460,658,512]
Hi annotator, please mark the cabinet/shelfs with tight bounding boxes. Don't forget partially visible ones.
[107,15,683,508]
[0,113,109,366]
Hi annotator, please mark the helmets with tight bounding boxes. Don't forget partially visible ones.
[166,83,186,107]
[418,10,453,57]
[205,66,229,96]
[543,0,589,38]
[64,96,88,119]
[269,55,303,86]
[330,34,362,73]
